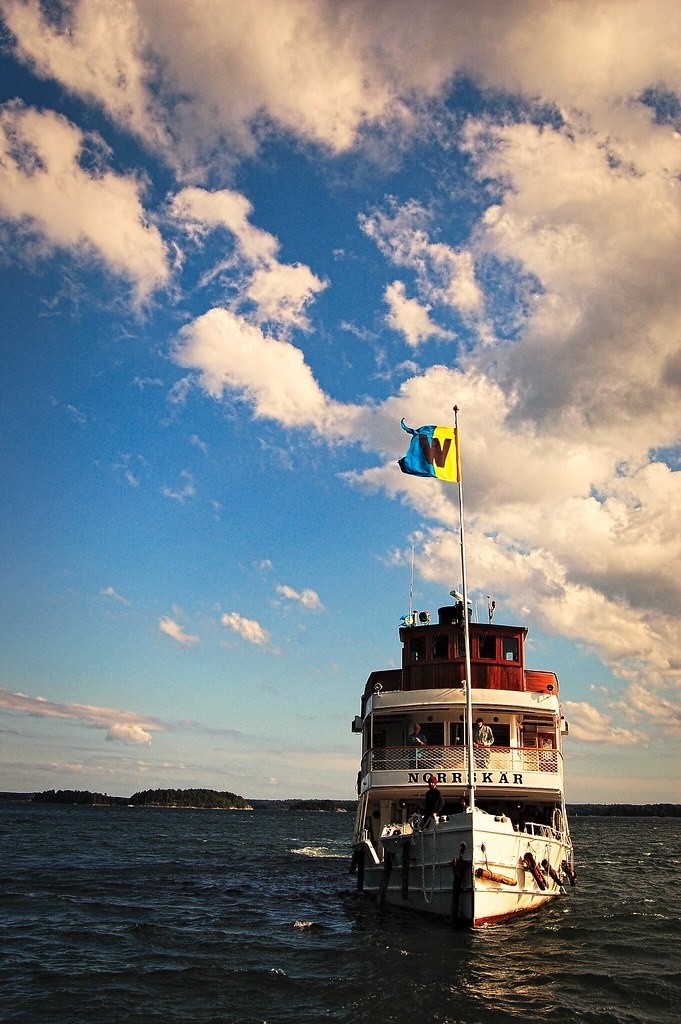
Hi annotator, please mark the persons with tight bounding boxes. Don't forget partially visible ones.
[458,796,469,813]
[407,723,427,769]
[424,776,445,827]
[473,718,494,769]
[543,739,552,749]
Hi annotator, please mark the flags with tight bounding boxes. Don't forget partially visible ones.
[397,418,459,483]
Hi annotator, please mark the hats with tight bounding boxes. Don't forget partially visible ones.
[428,776,437,785]
[475,718,483,722]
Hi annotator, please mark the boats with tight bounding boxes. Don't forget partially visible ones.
[351,405,575,930]
